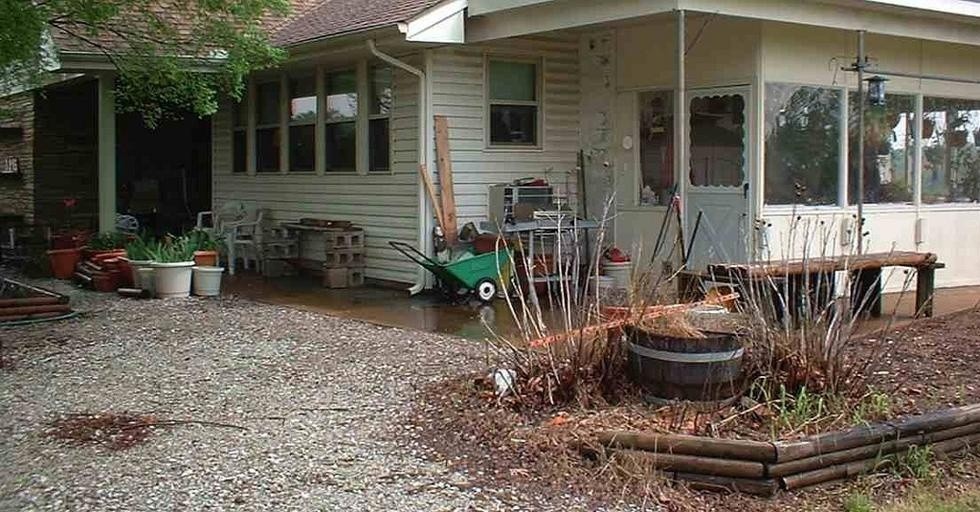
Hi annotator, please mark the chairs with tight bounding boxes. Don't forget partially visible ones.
[196,197,266,276]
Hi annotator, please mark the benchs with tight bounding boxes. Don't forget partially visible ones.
[706,249,945,332]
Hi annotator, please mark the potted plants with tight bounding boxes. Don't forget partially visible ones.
[121,226,232,300]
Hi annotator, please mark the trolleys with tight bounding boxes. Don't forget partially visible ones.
[389,240,511,305]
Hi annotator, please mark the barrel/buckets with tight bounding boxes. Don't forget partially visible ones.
[600,260,634,288]
[625,315,756,400]
[709,284,735,313]
[587,275,617,296]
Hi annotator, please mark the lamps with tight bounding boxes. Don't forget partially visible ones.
[859,60,891,107]
